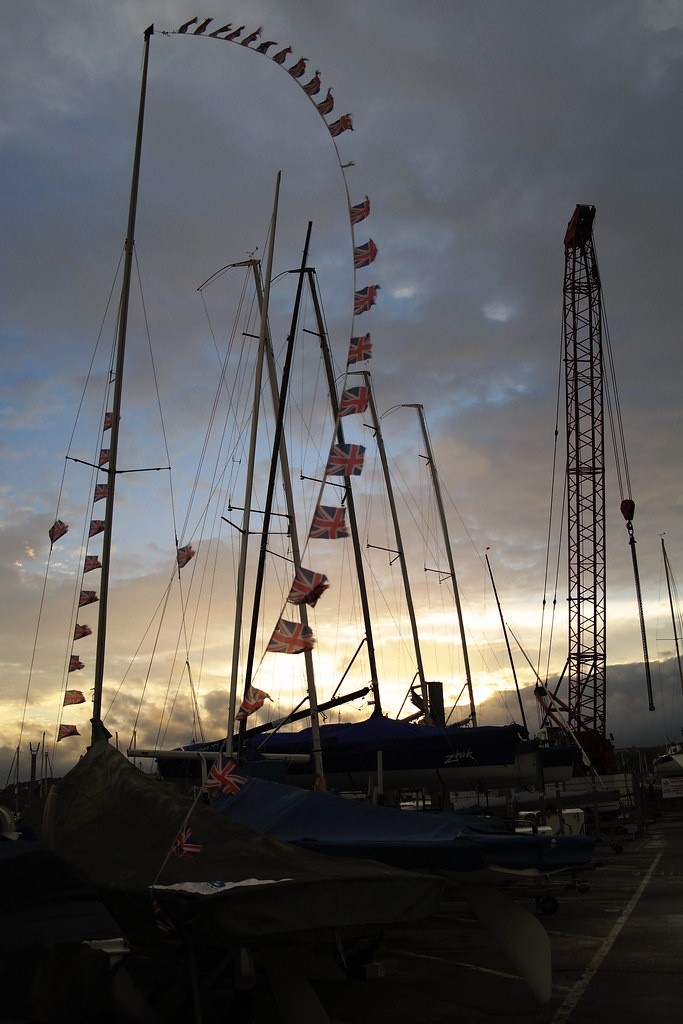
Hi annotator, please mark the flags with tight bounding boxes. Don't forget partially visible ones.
[348,335,371,366]
[204,754,250,798]
[350,199,370,226]
[170,820,208,860]
[148,890,173,940]
[354,284,378,317]
[178,17,355,137]
[339,386,372,417]
[286,565,327,608]
[309,503,350,540]
[324,444,365,475]
[235,688,269,723]
[46,413,124,739]
[267,618,315,654]
[178,545,195,569]
[353,239,378,270]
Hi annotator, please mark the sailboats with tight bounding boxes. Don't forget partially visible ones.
[0,24,683,1004]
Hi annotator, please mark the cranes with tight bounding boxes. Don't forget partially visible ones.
[535,204,655,775]
